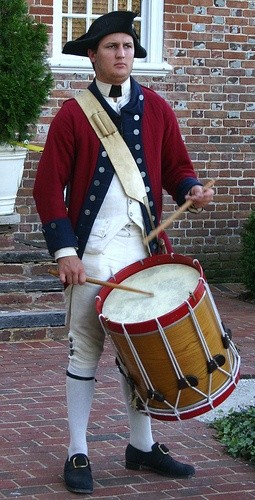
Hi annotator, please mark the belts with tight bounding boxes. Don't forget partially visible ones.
[117,225,142,237]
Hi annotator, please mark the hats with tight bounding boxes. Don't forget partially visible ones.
[62,11,147,58]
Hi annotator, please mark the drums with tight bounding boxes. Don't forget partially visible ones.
[93,252,241,423]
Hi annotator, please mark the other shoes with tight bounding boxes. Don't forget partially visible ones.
[64,453,93,493]
[125,441,195,480]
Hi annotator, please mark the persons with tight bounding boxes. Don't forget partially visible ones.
[31,10,216,494]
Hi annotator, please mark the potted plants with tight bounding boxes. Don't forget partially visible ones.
[0,0,54,217]
